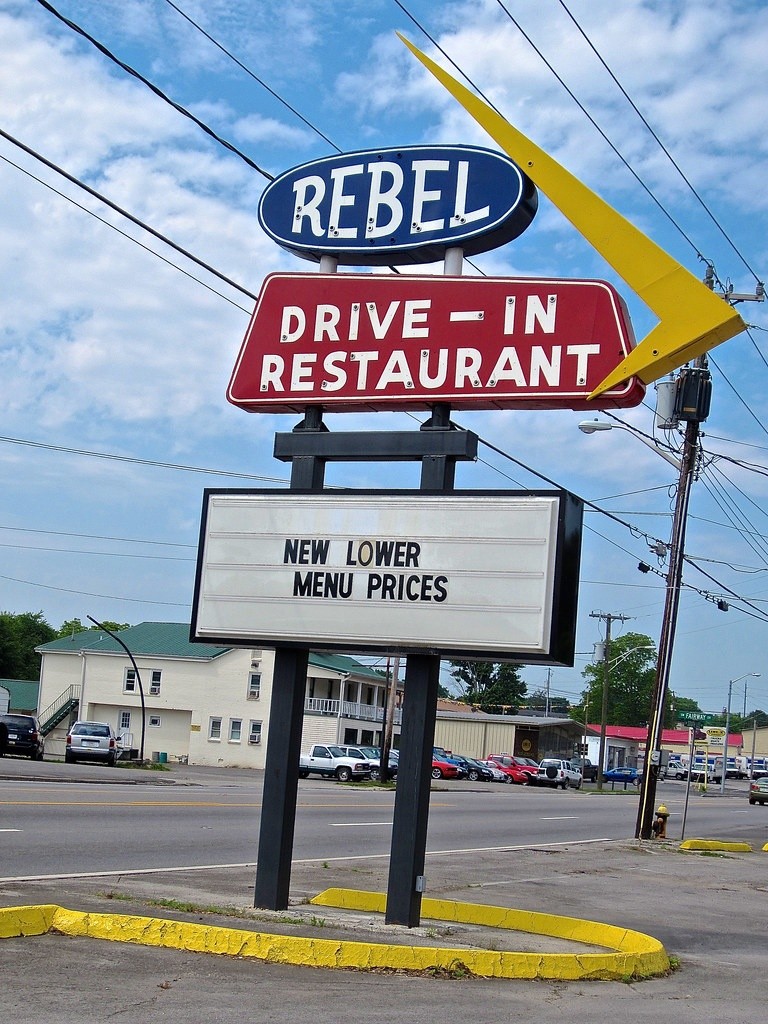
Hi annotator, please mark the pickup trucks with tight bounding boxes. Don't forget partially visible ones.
[571,758,599,783]
[300,744,369,782]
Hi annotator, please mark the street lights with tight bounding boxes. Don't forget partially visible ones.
[721,673,761,795]
[579,420,693,842]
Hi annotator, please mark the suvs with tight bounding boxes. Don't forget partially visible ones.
[0,714,44,761]
[65,720,121,765]
[538,759,582,790]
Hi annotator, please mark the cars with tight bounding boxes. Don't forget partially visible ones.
[749,777,768,805]
[602,767,643,785]
[339,746,398,781]
[376,747,538,788]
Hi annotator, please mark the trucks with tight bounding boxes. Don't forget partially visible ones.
[668,753,768,784]
